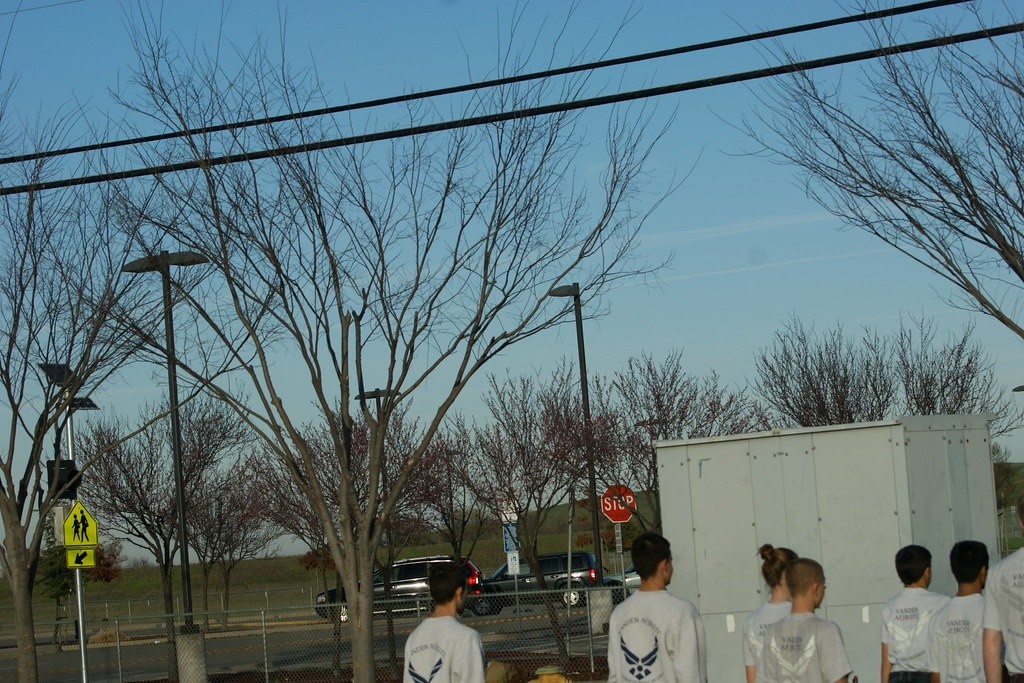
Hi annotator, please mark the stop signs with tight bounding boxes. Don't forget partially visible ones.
[600,483,638,524]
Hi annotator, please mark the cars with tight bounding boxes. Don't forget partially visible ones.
[603,561,642,605]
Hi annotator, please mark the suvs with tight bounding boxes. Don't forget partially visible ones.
[315,553,484,621]
[472,550,598,618]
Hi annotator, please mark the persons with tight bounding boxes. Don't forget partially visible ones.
[741,544,798,683]
[982,495,1024,683]
[756,558,853,683]
[880,545,951,683]
[403,563,486,683]
[608,533,707,683]
[928,541,989,683]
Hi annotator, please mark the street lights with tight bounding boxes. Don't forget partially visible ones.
[443,447,462,544]
[36,359,100,683]
[354,388,404,563]
[119,248,212,635]
[545,282,605,589]
